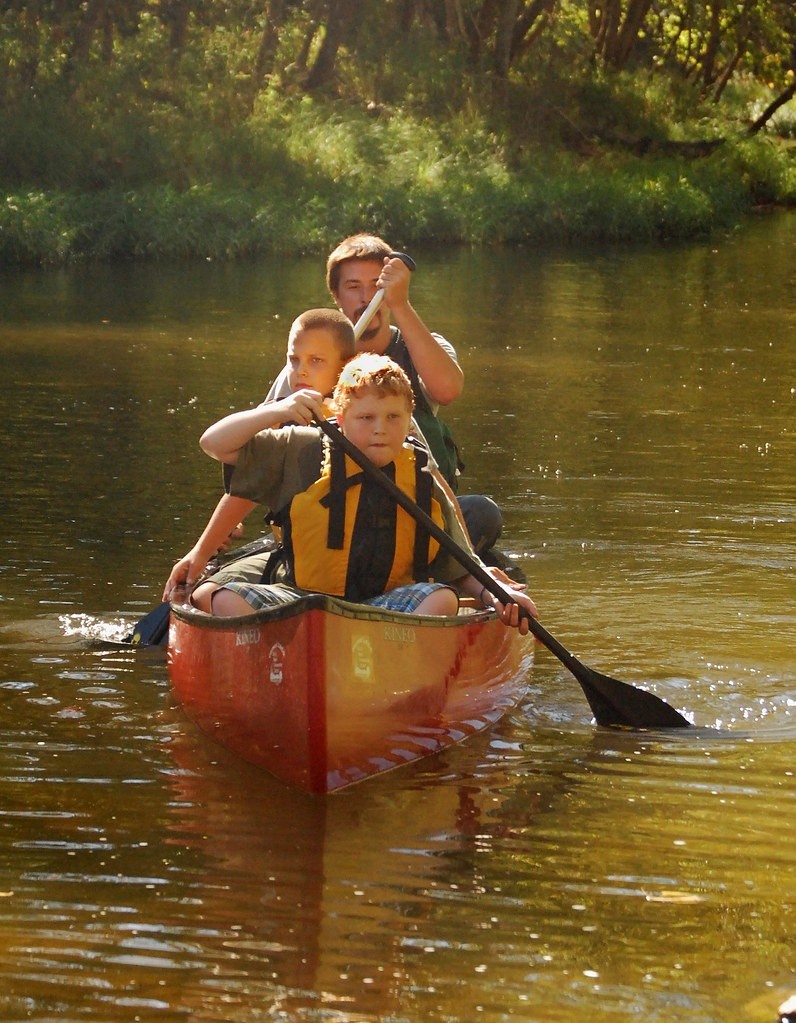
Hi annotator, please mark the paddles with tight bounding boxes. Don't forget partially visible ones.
[124,252,418,647]
[311,411,692,729]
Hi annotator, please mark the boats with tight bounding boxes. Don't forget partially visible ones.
[168,594,535,799]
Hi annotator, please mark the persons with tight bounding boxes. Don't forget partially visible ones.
[162,233,528,614]
[198,351,539,635]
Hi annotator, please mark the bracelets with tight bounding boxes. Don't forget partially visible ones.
[480,578,500,606]
